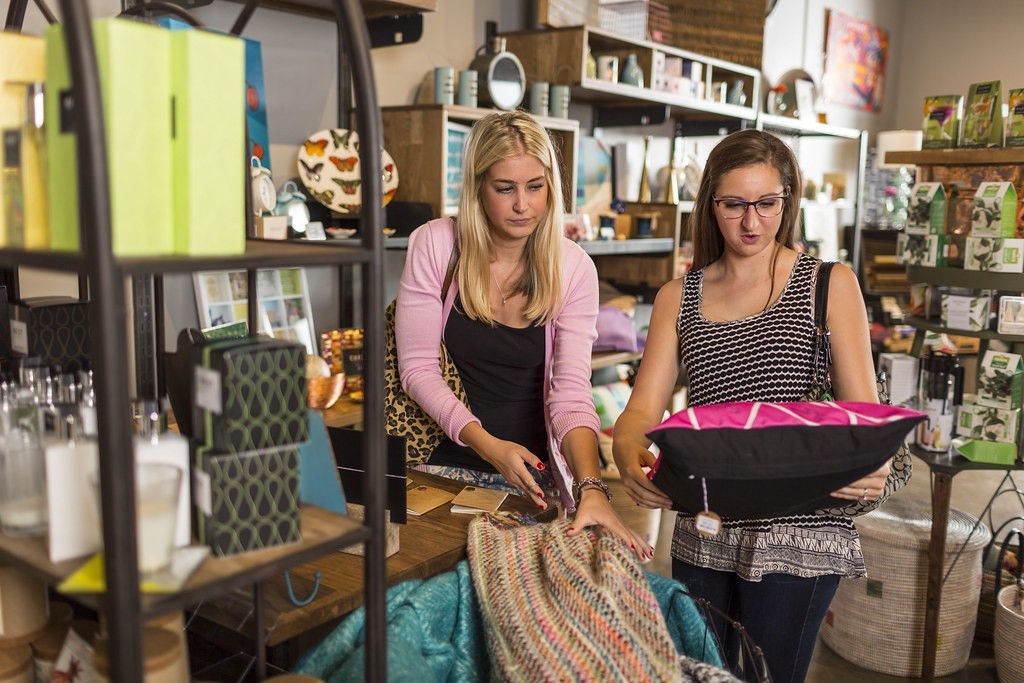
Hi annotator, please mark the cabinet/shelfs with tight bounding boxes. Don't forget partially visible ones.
[0,0,435,683]
[885,147,1024,682]
[353,25,869,374]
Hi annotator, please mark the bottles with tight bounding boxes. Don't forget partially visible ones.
[621,52,644,88]
[727,79,746,107]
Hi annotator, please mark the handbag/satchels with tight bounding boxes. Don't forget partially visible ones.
[383,215,463,470]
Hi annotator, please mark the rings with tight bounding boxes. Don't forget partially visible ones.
[517,488,525,497]
[863,488,868,500]
[527,480,538,488]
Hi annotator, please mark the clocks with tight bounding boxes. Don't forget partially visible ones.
[249,155,277,216]
[276,180,311,237]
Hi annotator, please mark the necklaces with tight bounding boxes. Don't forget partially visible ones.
[492,272,511,306]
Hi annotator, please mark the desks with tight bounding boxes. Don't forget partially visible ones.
[188,467,559,672]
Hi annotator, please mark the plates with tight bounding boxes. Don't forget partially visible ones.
[297,128,399,216]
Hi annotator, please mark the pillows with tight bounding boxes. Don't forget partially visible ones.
[591,282,647,434]
[645,401,928,515]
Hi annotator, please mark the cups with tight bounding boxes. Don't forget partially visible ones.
[551,85,570,119]
[434,66,454,105]
[457,69,477,109]
[597,56,619,84]
[918,373,955,452]
[2,358,166,446]
[529,81,548,116]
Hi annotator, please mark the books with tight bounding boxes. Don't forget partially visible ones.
[407,476,509,516]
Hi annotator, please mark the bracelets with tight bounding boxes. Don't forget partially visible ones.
[577,477,612,502]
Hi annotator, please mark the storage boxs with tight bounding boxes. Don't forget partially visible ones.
[251,215,288,240]
[877,182,1024,466]
[0,283,94,379]
[0,17,247,257]
[185,334,308,553]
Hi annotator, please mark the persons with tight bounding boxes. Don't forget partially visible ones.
[394,110,655,560]
[612,129,914,683]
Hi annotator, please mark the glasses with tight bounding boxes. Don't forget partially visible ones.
[709,191,794,220]
